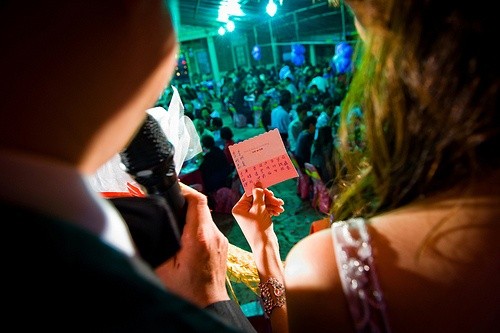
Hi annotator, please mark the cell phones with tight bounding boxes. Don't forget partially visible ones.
[107,197,182,269]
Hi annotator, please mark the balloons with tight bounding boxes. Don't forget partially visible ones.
[289,44,305,67]
[332,41,353,73]
[252,45,262,62]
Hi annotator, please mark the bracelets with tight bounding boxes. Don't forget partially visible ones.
[259,278,288,320]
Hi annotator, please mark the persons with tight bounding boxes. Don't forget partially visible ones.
[233,0,500,332]
[0,0,256,333]
[184,65,364,221]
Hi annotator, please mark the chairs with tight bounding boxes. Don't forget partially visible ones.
[155,62,364,215]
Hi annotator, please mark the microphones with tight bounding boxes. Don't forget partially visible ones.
[120,114,187,234]
